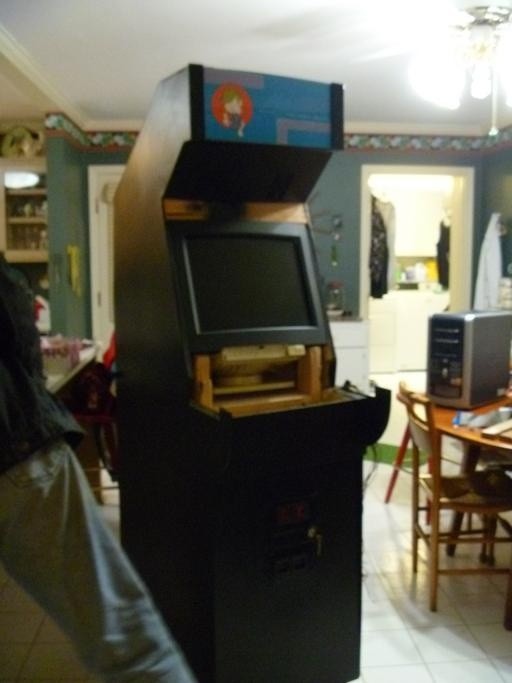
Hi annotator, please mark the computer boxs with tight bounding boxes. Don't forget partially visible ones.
[423,308,511,409]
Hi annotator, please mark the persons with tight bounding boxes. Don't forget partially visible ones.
[1,246,199,683]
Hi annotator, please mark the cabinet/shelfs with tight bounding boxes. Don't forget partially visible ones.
[0,176,49,262]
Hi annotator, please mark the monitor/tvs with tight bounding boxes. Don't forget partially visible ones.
[183,222,331,346]
